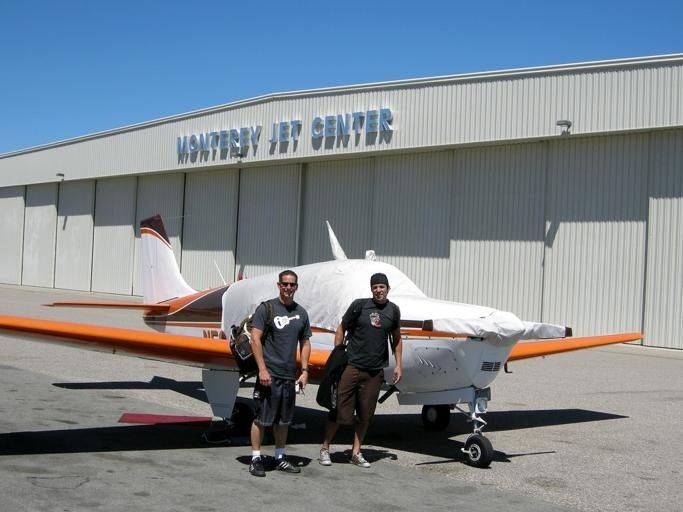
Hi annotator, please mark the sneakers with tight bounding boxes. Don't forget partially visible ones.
[271,457,301,473]
[249,459,266,477]
[319,449,332,466]
[349,452,371,469]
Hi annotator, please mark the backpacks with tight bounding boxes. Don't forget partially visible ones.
[229,300,274,375]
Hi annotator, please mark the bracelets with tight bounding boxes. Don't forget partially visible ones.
[300,368,309,372]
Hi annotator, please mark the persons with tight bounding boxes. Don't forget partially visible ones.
[319,273,402,468]
[248,270,312,477]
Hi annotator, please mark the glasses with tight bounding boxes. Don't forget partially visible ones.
[280,282,297,288]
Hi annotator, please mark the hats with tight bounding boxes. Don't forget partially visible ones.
[371,273,388,286]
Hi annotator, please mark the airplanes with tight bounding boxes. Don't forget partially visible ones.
[0,214,648,467]
